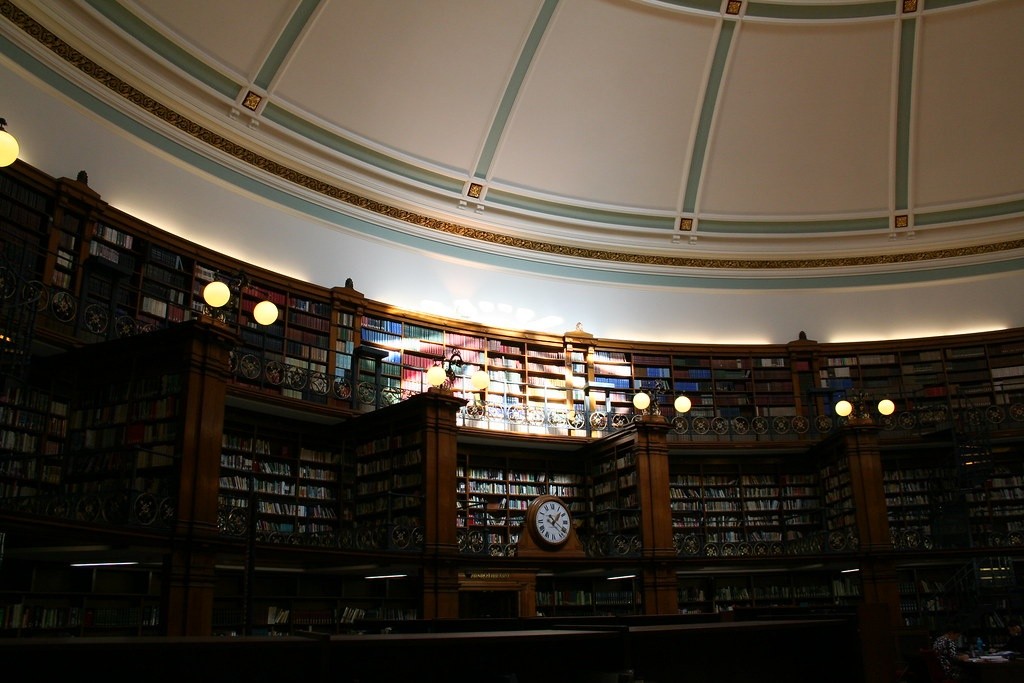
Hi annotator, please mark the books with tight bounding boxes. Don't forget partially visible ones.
[0,215,1024,663]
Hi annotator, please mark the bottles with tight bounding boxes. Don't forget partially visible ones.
[976,637,984,657]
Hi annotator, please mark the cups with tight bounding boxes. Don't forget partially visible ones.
[1007,653,1015,663]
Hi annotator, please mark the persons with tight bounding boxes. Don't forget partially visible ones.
[932,619,1024,683]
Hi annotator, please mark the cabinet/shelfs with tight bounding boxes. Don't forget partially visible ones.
[0,161,1024,625]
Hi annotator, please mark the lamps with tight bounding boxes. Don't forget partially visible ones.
[202,265,279,329]
[632,380,691,415]
[835,389,895,418]
[0,116,17,168]
[426,347,490,392]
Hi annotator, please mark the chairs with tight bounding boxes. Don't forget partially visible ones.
[919,648,958,683]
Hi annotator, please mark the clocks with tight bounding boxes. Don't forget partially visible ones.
[527,494,573,550]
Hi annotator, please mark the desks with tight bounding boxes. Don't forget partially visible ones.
[959,656,1024,683]
